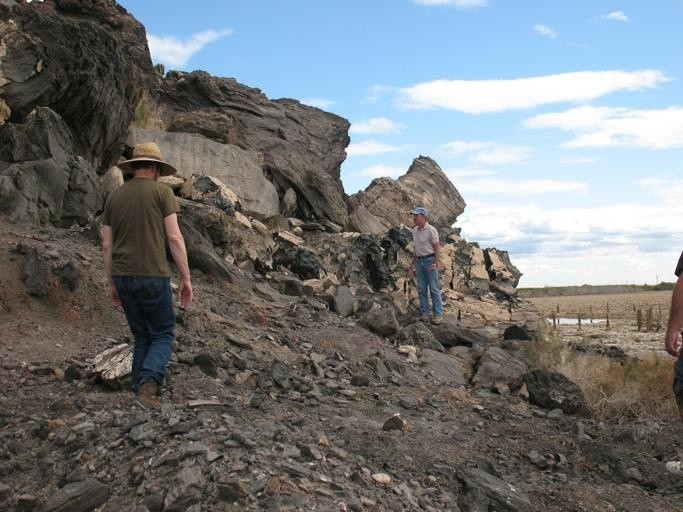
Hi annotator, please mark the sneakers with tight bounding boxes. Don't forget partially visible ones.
[665,460,682,475]
[419,315,443,324]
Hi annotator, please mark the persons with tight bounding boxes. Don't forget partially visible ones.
[661,250,682,477]
[403,205,443,326]
[98,142,193,413]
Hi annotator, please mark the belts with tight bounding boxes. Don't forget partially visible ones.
[417,253,435,259]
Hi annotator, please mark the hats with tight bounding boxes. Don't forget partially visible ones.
[116,142,176,178]
[409,207,427,216]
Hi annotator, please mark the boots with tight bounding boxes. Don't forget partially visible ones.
[131,379,162,409]
